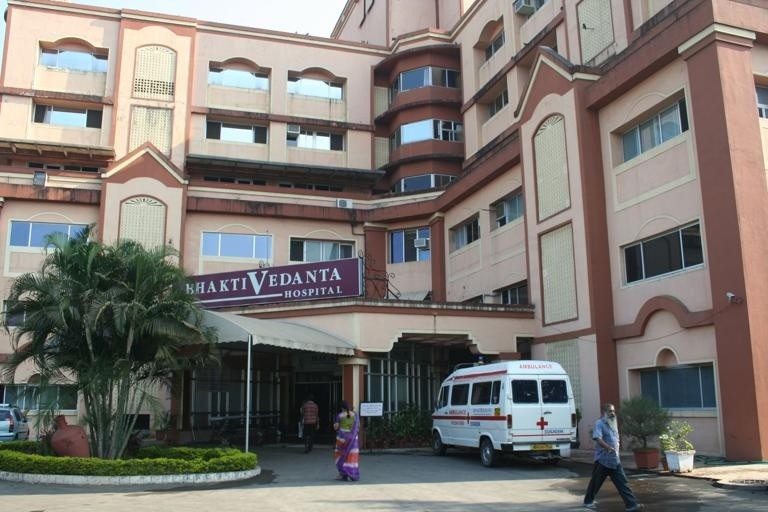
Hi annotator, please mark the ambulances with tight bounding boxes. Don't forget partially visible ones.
[430,359,579,467]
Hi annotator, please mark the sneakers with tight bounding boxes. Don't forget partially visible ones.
[624,503,645,512]
[583,502,597,509]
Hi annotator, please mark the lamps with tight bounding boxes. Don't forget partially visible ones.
[32,171,48,189]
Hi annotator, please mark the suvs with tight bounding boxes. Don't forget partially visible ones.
[0,403,30,443]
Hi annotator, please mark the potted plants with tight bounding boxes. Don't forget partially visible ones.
[618,393,695,473]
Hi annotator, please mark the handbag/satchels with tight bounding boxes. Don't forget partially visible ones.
[297,419,304,438]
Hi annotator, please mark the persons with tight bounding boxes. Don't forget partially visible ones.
[580,402,647,512]
[298,393,320,454]
[332,399,360,483]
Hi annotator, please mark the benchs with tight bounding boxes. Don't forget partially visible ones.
[208,410,288,447]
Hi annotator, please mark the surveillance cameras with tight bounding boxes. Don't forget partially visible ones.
[727,292,734,302]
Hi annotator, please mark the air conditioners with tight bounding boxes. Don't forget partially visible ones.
[413,238,427,248]
[513,0,535,16]
[288,124,300,139]
[336,199,353,209]
